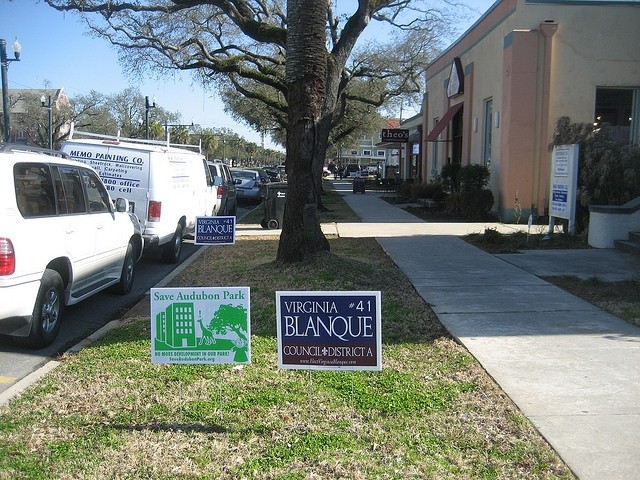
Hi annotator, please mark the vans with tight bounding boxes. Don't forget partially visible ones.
[207,159,242,216]
[61,139,222,264]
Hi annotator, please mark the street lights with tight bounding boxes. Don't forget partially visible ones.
[40,94,53,155]
[145,96,156,140]
[0,38,22,143]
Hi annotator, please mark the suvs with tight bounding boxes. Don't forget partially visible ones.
[0,142,145,350]
[343,164,360,179]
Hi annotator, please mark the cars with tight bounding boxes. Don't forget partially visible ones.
[248,168,271,182]
[263,167,281,182]
[277,165,286,173]
[229,168,262,205]
[322,166,331,177]
[365,164,378,171]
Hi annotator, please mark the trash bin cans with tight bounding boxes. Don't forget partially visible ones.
[261,181,288,229]
